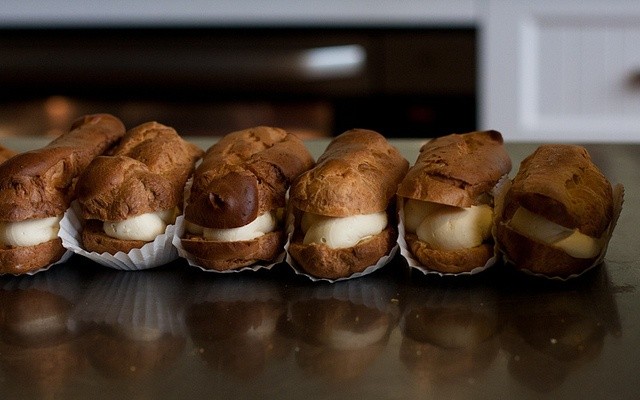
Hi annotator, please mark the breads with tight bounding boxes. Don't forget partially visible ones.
[184,303,293,380]
[0,291,74,400]
[0,112,127,275]
[77,320,177,383]
[400,308,506,382]
[179,125,315,270]
[496,144,613,276]
[288,299,395,386]
[289,128,409,279]
[394,129,512,272]
[74,121,206,256]
[501,314,607,390]
